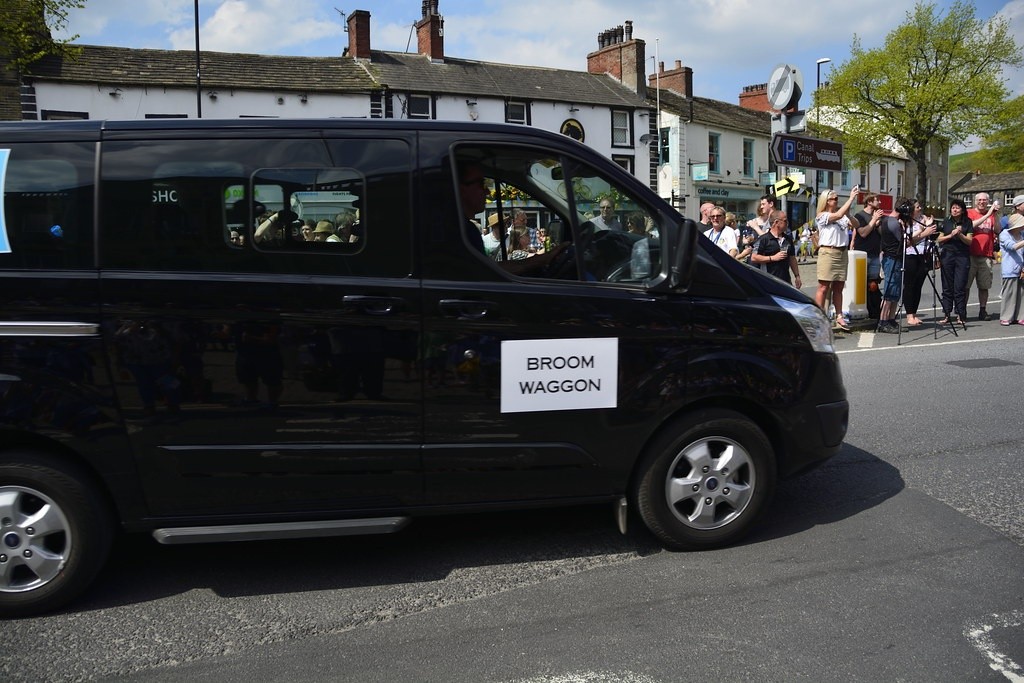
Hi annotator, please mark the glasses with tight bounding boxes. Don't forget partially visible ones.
[709,214,725,218]
[827,197,838,201]
[600,206,615,209]
[302,228,310,232]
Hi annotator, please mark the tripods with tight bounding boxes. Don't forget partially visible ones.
[874,214,966,345]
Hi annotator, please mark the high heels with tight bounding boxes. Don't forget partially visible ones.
[836,320,849,330]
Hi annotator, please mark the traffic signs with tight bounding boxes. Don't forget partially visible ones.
[770,131,843,173]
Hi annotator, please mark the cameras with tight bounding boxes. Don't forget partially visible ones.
[895,204,910,214]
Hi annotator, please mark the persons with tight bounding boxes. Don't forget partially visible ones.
[696,194,802,290]
[855,193,884,317]
[1001,195,1024,239]
[935,199,973,326]
[967,192,1000,320]
[999,213,1024,326]
[877,197,916,334]
[902,198,937,326]
[225,211,360,247]
[815,183,860,332]
[482,202,662,264]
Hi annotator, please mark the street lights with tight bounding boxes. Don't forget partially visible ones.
[816,57,832,208]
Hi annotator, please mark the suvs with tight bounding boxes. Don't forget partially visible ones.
[0,118,849,620]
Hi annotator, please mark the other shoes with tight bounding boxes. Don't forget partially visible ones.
[914,317,923,324]
[1014,320,1024,324]
[939,316,950,323]
[888,322,909,332]
[876,322,900,334]
[907,317,918,324]
[959,313,967,321]
[978,312,992,322]
[1000,320,1010,326]
[956,316,965,324]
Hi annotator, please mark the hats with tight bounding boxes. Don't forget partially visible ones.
[1007,213,1024,231]
[1012,194,1024,207]
[312,221,333,233]
[486,213,509,227]
